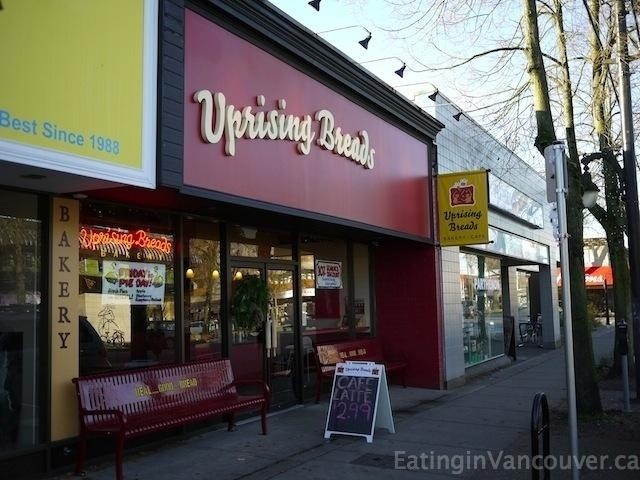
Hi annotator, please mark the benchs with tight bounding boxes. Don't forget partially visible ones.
[72,359,271,479]
[314,338,406,404]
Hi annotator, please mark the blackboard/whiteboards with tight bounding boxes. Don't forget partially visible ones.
[323,360,395,443]
